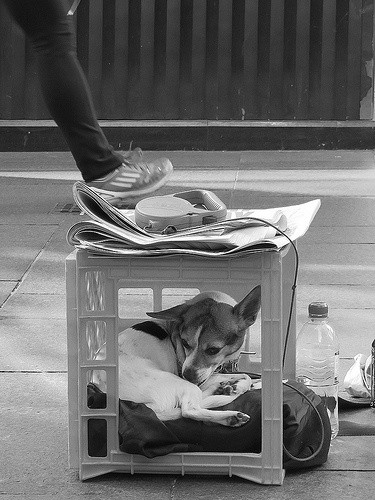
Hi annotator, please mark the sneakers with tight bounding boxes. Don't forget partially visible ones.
[89,158,174,203]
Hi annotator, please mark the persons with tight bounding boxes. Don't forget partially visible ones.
[1,1,177,203]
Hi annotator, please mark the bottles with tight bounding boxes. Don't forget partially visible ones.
[296,302,339,441]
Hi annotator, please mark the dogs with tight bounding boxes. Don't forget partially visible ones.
[90,283,261,428]
